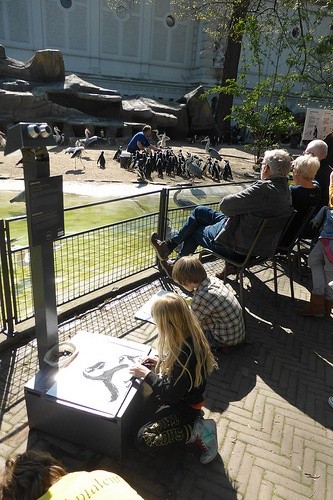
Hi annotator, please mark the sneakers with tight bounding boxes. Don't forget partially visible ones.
[160,257,174,280]
[194,419,218,464]
[149,233,172,261]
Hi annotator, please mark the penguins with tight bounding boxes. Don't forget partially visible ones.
[113,144,234,182]
[97,151,107,168]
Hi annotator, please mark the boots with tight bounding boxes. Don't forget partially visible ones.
[295,293,326,318]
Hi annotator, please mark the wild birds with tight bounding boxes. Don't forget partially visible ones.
[201,136,223,161]
[51,126,245,144]
[0,131,11,163]
[9,191,26,208]
[63,128,91,170]
[169,183,199,211]
[15,158,23,166]
[186,182,208,201]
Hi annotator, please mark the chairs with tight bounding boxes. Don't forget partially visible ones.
[198,210,297,330]
[236,204,327,302]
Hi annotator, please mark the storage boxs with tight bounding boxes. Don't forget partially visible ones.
[24,331,162,462]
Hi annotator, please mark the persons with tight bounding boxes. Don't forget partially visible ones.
[279,154,322,248]
[293,205,333,318]
[328,171,333,210]
[172,254,246,351]
[129,293,218,464]
[303,140,333,206]
[0,450,146,500]
[322,129,333,168]
[127,125,160,154]
[150,149,292,280]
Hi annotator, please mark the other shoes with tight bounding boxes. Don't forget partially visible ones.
[136,167,144,177]
